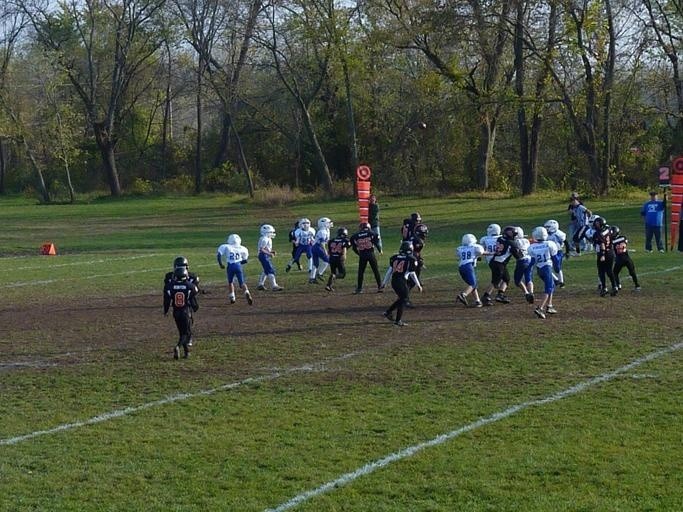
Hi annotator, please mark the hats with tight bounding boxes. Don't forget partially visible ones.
[650,192,658,196]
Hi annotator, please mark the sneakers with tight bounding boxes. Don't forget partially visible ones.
[555,278,565,289]
[525,290,557,319]
[229,295,236,303]
[645,248,665,254]
[405,302,416,308]
[352,289,363,294]
[172,338,193,359]
[458,290,513,309]
[245,292,253,306]
[569,247,593,257]
[285,263,336,292]
[377,288,384,293]
[258,285,283,291]
[383,311,408,326]
[598,283,641,297]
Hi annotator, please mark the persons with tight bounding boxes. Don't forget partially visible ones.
[380,211,430,329]
[456,233,500,309]
[162,265,200,363]
[368,194,383,256]
[640,188,669,255]
[285,215,335,286]
[216,232,253,307]
[349,222,384,294]
[479,218,571,320]
[324,228,352,293]
[163,255,201,348]
[257,223,285,292]
[567,191,643,297]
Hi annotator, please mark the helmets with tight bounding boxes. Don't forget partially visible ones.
[359,223,371,233]
[412,214,427,240]
[399,241,414,255]
[594,217,620,240]
[486,223,501,235]
[298,219,311,231]
[570,193,578,201]
[317,217,334,230]
[227,233,241,245]
[462,234,477,245]
[503,226,523,240]
[174,257,189,282]
[337,228,347,237]
[260,224,276,238]
[532,227,548,241]
[544,219,559,233]
